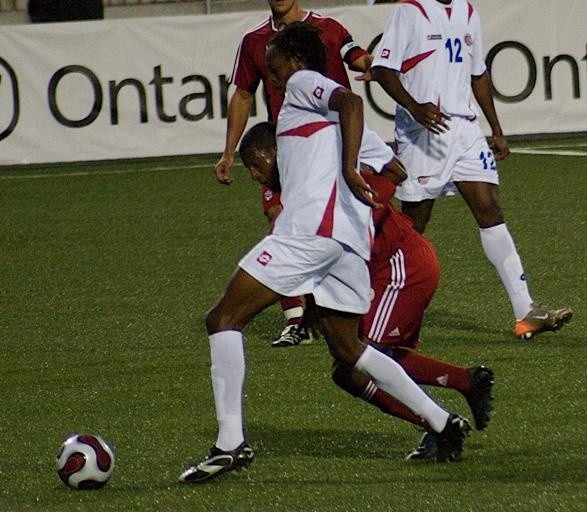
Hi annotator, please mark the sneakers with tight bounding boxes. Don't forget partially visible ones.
[271,323,314,348]
[404,412,474,462]
[463,365,496,432]
[178,440,255,484]
[514,302,575,340]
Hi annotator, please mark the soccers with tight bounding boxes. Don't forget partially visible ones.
[56,434,115,489]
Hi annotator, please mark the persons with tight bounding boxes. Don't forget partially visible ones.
[241,122,491,457]
[371,0,573,346]
[174,19,471,484]
[215,0,377,347]
